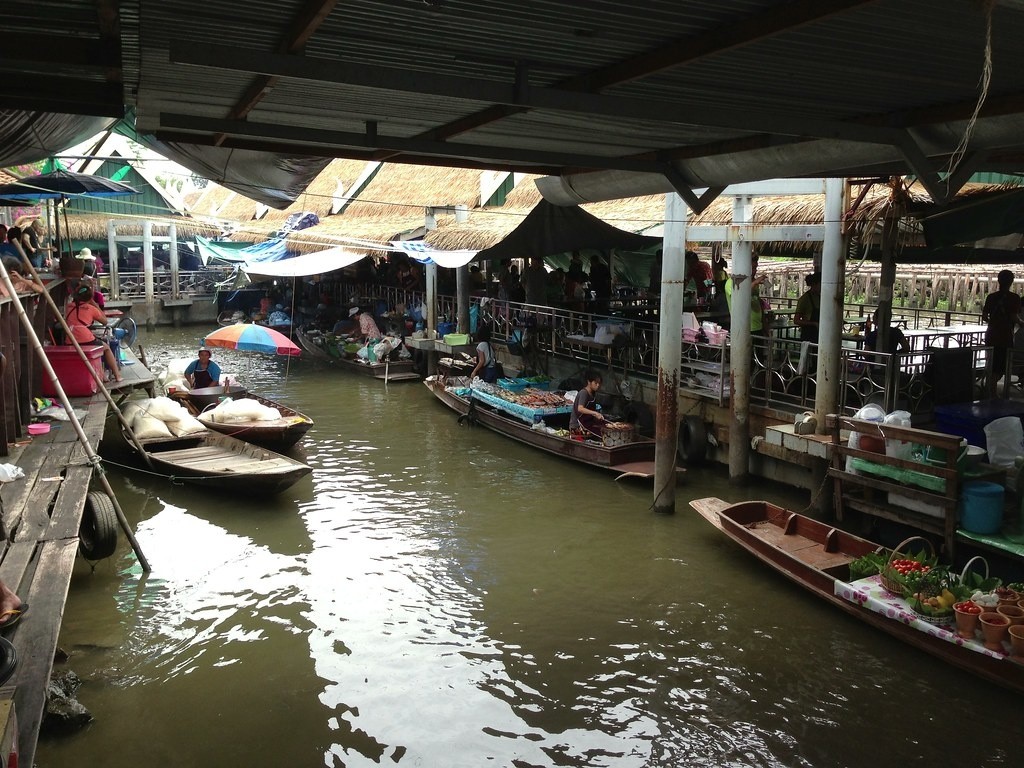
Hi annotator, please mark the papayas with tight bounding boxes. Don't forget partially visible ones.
[936,588,956,609]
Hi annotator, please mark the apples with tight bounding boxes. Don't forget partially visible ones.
[956,600,980,613]
[892,559,930,574]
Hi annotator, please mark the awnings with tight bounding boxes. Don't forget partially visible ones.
[240,244,369,276]
[195,211,319,289]
[389,239,480,268]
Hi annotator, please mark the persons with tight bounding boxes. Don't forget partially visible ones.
[291,253,483,304]
[851,306,910,367]
[61,246,203,294]
[982,270,1024,400]
[470,326,498,385]
[794,271,821,388]
[647,247,768,373]
[64,285,123,382]
[348,307,381,345]
[497,251,612,331]
[569,370,605,437]
[259,297,290,325]
[184,347,219,391]
[0,218,57,293]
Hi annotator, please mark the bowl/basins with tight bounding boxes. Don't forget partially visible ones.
[188,386,246,412]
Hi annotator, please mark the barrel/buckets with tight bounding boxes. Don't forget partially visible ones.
[925,442,986,471]
[959,479,1005,534]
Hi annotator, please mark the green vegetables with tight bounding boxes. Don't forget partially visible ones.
[525,373,553,382]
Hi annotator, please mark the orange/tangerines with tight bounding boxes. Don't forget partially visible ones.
[1008,582,1024,593]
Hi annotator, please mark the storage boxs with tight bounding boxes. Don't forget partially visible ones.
[497,378,528,392]
[522,378,550,391]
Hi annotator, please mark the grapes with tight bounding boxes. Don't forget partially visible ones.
[913,579,940,599]
[992,587,1016,600]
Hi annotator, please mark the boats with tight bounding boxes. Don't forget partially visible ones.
[169,376,315,454]
[119,399,317,505]
[295,324,421,383]
[688,496,1024,697]
[425,369,689,478]
[217,310,296,340]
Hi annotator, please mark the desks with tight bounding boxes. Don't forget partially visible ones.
[90,318,120,329]
[105,309,123,317]
[39,345,104,396]
[597,296,988,373]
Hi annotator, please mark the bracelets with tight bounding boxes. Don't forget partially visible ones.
[29,283,35,288]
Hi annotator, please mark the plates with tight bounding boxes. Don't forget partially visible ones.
[28,423,50,434]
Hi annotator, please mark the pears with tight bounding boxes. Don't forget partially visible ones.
[900,570,924,583]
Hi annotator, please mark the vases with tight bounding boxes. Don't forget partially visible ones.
[162,297,193,306]
[104,299,132,311]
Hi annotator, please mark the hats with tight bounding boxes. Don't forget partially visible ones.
[349,307,359,317]
[198,346,212,358]
[75,247,96,260]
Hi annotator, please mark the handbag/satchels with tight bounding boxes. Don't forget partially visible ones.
[880,536,989,625]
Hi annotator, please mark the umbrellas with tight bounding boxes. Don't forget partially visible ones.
[0,168,143,257]
[199,318,301,398]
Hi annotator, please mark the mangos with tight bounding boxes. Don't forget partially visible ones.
[913,592,938,611]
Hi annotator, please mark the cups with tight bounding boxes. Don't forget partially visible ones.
[952,587,1024,664]
[168,386,176,392]
